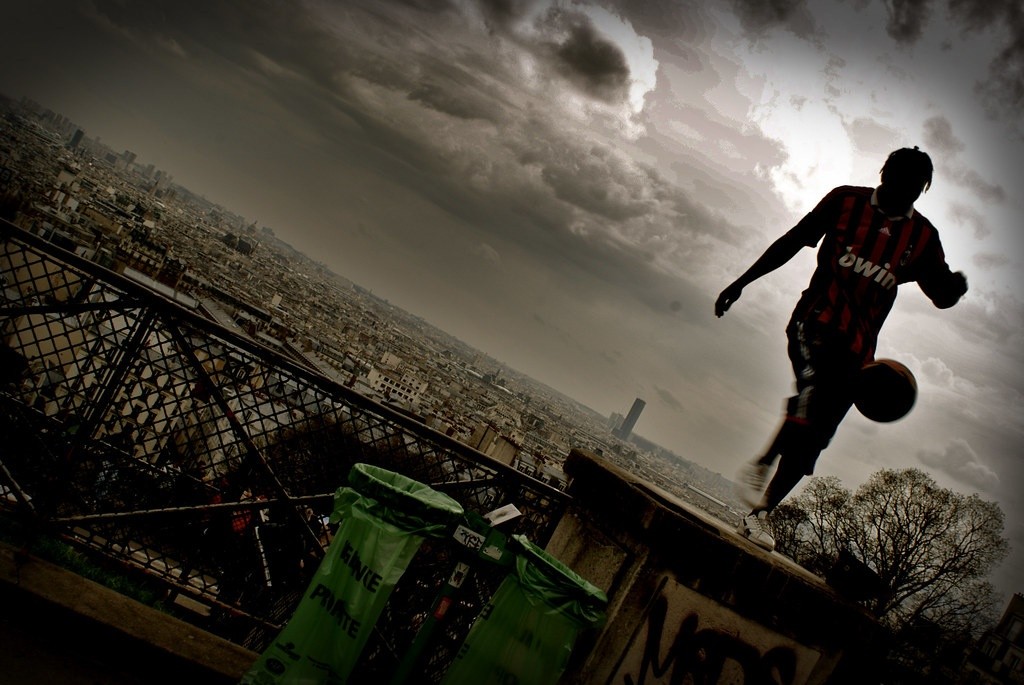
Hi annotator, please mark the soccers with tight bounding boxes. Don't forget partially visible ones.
[851,355,920,424]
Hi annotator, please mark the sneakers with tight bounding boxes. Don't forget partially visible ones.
[739,453,771,508]
[738,510,776,551]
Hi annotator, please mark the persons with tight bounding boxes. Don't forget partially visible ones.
[716,148,968,551]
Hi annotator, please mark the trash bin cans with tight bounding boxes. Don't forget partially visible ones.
[246,457,606,685]
